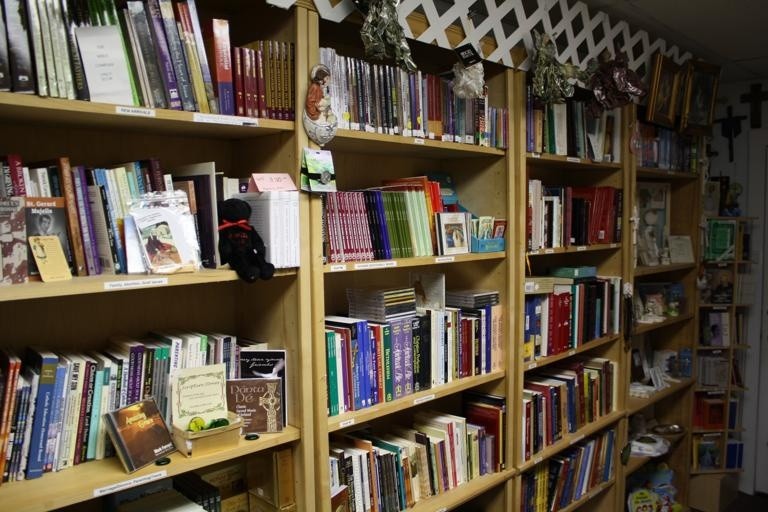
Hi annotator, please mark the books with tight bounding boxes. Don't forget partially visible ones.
[322,175,506,265]
[323,268,504,416]
[525,84,623,251]
[638,122,699,173]
[321,46,509,151]
[2,332,297,512]
[522,265,621,462]
[2,151,301,270]
[2,0,292,122]
[520,427,613,512]
[329,391,508,512]
[692,218,756,468]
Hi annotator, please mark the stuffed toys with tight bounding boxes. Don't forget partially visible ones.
[216,195,276,281]
